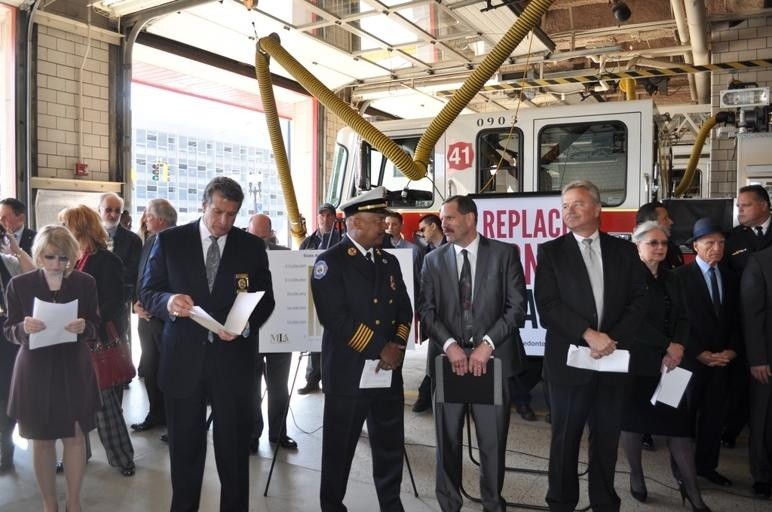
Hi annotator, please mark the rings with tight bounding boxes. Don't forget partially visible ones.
[454,364,460,368]
[173,311,179,317]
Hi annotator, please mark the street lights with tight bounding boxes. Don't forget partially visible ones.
[249,171,262,213]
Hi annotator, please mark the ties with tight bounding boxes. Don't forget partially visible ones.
[581,238,606,332]
[205,235,221,295]
[754,225,765,238]
[459,250,472,310]
[708,267,721,313]
[365,252,375,266]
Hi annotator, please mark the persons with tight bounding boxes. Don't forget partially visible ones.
[418,196,527,512]
[140,178,274,512]
[136,209,155,247]
[622,219,712,512]
[656,215,737,490]
[634,201,684,269]
[417,216,447,271]
[243,215,297,449]
[534,180,652,512]
[310,187,412,512]
[0,224,39,474]
[56,204,135,476]
[118,210,132,231]
[130,199,177,442]
[0,198,39,257]
[724,184,772,299]
[3,223,106,512]
[740,239,772,500]
[299,203,342,251]
[380,209,417,252]
[98,191,142,303]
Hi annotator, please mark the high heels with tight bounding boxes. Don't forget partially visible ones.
[679,484,713,512]
[54,458,66,472]
[118,462,136,478]
[628,472,650,502]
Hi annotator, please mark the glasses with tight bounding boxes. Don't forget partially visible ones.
[101,207,122,214]
[40,254,68,261]
[644,240,669,246]
[418,224,432,232]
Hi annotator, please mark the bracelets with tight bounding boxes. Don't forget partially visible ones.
[13,247,23,258]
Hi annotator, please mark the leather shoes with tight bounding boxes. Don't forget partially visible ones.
[412,392,431,414]
[456,341,478,348]
[269,435,298,451]
[298,381,321,395]
[161,433,171,443]
[130,420,161,431]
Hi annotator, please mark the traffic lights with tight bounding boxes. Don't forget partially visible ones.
[152,163,160,182]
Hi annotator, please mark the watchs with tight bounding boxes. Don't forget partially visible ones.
[482,339,494,354]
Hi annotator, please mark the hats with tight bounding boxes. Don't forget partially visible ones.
[318,203,335,214]
[685,215,729,244]
[338,185,394,219]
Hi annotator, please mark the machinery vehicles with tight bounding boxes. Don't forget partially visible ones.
[316,89,772,361]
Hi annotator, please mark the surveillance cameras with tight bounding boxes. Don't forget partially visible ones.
[603,0,632,24]
